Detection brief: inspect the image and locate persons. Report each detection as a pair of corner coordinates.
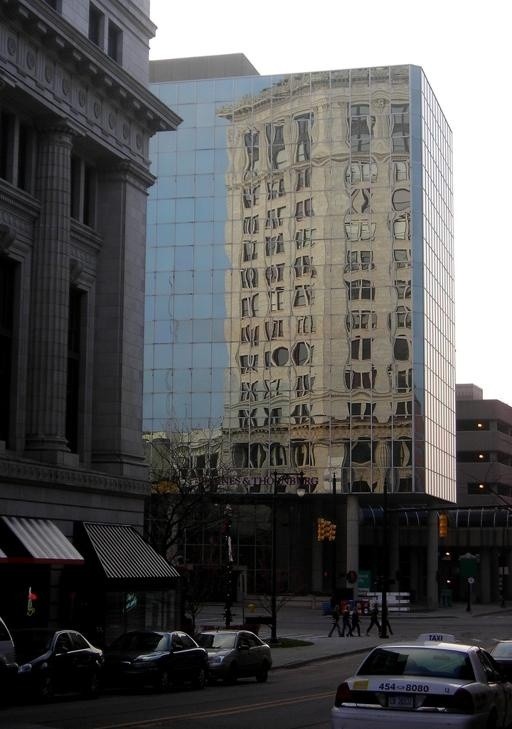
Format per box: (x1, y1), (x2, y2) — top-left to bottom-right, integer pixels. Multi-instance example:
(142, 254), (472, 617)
(366, 602), (382, 636)
(377, 605), (394, 635)
(346, 607), (361, 637)
(341, 604), (353, 637)
(325, 604), (343, 637)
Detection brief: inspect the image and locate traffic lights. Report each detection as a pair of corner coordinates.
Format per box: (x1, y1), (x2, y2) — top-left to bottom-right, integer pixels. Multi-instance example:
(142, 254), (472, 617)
(438, 514), (447, 538)
(325, 520), (332, 538)
(318, 518), (325, 541)
(329, 524), (336, 542)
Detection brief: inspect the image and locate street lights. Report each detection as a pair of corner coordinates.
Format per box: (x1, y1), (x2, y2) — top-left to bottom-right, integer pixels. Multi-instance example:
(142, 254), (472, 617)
(222, 503), (235, 627)
(270, 468), (309, 641)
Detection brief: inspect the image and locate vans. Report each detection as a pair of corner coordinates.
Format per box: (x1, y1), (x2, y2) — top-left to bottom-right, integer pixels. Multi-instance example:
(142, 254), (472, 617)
(0, 616), (16, 662)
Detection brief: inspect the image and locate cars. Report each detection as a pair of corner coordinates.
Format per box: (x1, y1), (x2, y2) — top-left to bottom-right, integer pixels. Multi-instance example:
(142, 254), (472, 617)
(0, 630), (104, 700)
(331, 633), (512, 729)
(193, 630), (272, 685)
(490, 640), (512, 678)
(104, 630), (208, 693)
